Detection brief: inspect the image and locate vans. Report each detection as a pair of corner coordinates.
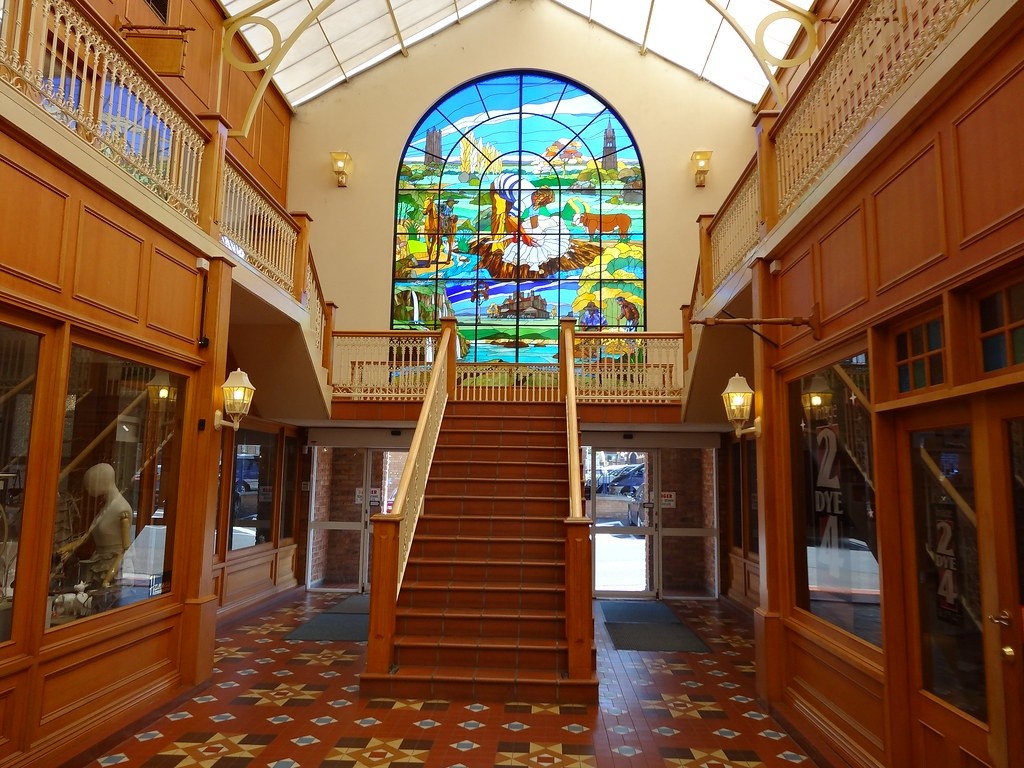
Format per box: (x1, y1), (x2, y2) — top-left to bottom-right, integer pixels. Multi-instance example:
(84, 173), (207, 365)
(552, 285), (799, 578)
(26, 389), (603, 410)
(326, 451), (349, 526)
(608, 462), (645, 497)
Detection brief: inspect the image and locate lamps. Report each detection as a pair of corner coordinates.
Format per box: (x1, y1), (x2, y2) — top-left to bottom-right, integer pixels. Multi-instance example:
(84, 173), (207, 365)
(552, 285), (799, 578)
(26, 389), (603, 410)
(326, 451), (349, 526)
(214, 366), (256, 431)
(721, 372), (761, 440)
(146, 371), (178, 426)
(691, 150), (712, 186)
(800, 374), (834, 429)
(329, 145), (353, 187)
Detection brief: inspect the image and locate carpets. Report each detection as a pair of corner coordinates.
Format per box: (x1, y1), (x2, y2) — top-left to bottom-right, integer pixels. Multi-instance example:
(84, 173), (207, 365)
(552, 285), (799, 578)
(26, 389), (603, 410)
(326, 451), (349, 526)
(281, 614), (370, 641)
(323, 593), (370, 613)
(600, 600), (681, 622)
(604, 622), (713, 652)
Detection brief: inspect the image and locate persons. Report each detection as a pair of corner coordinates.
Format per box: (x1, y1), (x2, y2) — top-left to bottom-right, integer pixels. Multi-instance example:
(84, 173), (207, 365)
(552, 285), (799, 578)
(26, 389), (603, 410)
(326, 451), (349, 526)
(55, 464), (134, 616)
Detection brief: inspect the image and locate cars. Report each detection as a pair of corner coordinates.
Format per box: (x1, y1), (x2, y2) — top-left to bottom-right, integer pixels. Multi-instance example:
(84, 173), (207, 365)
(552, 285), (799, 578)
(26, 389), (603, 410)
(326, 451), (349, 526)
(626, 483), (647, 536)
(217, 454), (259, 521)
